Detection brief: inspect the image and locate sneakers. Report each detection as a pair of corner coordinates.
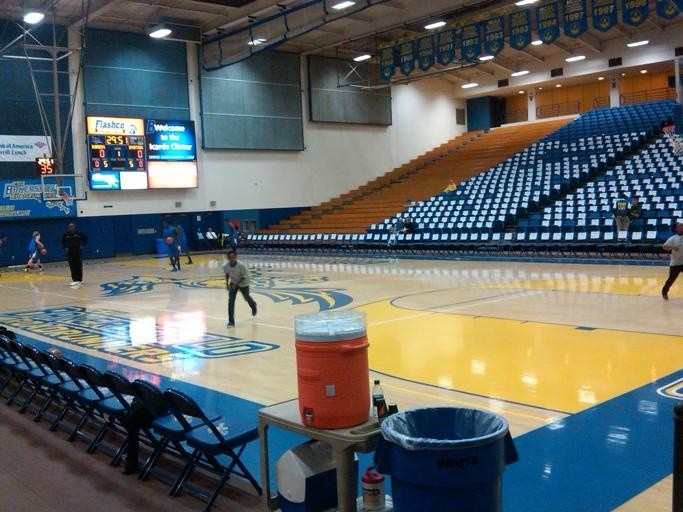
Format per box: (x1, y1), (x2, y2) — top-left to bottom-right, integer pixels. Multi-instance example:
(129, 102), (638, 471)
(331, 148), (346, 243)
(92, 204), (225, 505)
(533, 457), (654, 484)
(227, 321), (235, 328)
(69, 281), (79, 286)
(251, 301), (257, 317)
(661, 290), (669, 300)
(171, 267), (177, 272)
(188, 260), (192, 264)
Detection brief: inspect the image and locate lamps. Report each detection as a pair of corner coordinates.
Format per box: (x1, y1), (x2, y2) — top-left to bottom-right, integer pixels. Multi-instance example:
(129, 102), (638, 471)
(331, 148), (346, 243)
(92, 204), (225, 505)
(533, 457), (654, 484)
(515, 0), (539, 6)
(424, 20), (446, 32)
(145, 4), (173, 40)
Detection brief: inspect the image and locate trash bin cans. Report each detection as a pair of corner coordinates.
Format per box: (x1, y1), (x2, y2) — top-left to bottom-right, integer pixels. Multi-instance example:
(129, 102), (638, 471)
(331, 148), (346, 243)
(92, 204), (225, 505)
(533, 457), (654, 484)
(376, 403), (519, 512)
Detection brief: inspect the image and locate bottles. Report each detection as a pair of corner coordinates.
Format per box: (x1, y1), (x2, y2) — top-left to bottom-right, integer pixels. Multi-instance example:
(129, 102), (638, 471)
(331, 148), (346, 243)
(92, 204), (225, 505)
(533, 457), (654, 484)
(372, 379), (384, 418)
(361, 471), (385, 511)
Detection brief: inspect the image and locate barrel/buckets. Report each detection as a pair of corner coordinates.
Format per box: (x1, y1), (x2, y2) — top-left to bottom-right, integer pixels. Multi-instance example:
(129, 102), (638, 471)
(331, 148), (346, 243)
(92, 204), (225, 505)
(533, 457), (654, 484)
(294, 311), (369, 429)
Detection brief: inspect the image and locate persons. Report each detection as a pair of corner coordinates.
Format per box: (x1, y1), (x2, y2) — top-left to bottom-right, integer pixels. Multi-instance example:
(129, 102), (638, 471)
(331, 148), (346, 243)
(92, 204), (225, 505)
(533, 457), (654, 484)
(196, 218), (241, 251)
(222, 251), (256, 328)
(395, 218), (405, 232)
(443, 180), (457, 193)
(661, 222), (683, 300)
(612, 194), (631, 243)
(22, 231), (44, 272)
(404, 218), (416, 232)
(223, 219), (241, 251)
(161, 220), (193, 272)
(629, 196), (642, 219)
(62, 221), (88, 287)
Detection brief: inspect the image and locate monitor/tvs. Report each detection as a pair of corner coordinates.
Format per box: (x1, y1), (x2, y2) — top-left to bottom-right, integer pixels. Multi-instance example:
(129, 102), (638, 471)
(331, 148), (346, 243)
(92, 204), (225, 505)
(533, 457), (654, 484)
(143, 118), (197, 161)
(148, 161), (200, 189)
(119, 171), (148, 190)
(88, 172), (120, 190)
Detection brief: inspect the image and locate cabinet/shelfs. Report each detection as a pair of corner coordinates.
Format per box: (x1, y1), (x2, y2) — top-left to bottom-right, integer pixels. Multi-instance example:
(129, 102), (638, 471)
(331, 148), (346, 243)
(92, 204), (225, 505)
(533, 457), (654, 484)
(257, 398), (388, 512)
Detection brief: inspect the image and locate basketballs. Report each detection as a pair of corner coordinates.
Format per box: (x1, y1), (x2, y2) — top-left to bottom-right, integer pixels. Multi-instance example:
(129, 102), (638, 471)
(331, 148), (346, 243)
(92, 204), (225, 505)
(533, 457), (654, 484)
(48, 348), (62, 359)
(167, 237), (173, 244)
(41, 249), (46, 255)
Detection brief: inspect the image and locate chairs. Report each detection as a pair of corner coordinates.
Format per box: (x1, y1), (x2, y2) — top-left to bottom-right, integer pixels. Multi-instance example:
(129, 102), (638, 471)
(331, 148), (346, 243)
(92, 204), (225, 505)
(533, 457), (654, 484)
(263, 235), (268, 250)
(345, 234), (351, 252)
(389, 234), (396, 255)
(69, 363), (112, 453)
(100, 369), (162, 487)
(16, 345), (54, 413)
(589, 231), (601, 258)
(616, 230), (629, 259)
(291, 235), (297, 249)
(413, 234), (422, 255)
(257, 234), (262, 249)
(35, 351), (71, 433)
(431, 233), (441, 256)
(127, 378), (220, 502)
(644, 231), (658, 260)
(513, 233), (526, 257)
(337, 234), (344, 251)
(323, 235), (330, 251)
(539, 233), (551, 259)
(6, 339), (45, 403)
(366, 233), (374, 254)
(564, 232), (576, 257)
(280, 235), (285, 249)
(374, 234), (381, 253)
(397, 234), (405, 255)
(441, 234), (449, 255)
(252, 234), (257, 250)
(310, 235), (316, 250)
(316, 234), (323, 251)
(450, 234), (458, 255)
(489, 233), (501, 257)
(359, 233), (366, 254)
(526, 233), (539, 257)
(469, 233), (478, 257)
(162, 389), (264, 509)
(576, 232), (588, 259)
(423, 232), (432, 256)
(459, 233), (469, 256)
(479, 233), (490, 256)
(244, 235), (252, 249)
(0, 335), (31, 398)
(274, 235), (279, 249)
(406, 233), (413, 255)
(269, 235), (274, 250)
(501, 233), (513, 257)
(601, 232), (615, 258)
(352, 234), (359, 253)
(285, 234), (291, 249)
(303, 235), (309, 250)
(297, 235), (303, 250)
(552, 232), (562, 257)
(381, 234), (389, 254)
(331, 234), (337, 251)
(52, 358), (91, 445)
(629, 232), (644, 259)
